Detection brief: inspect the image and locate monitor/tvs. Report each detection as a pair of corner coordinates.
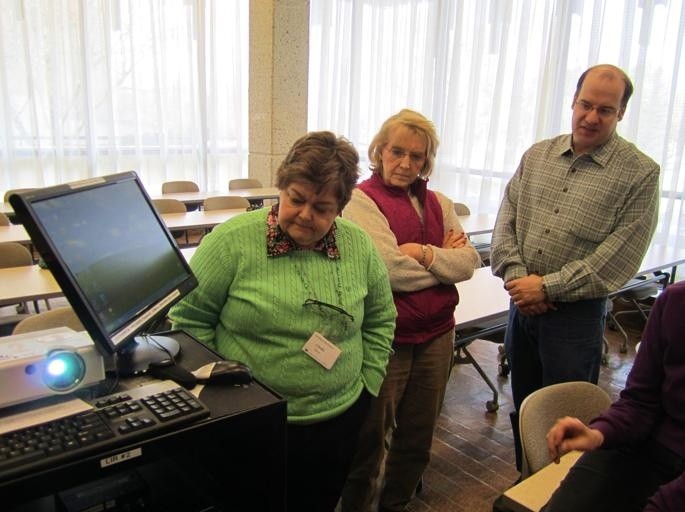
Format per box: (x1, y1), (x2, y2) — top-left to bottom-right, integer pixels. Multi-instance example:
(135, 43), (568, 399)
(0, 325), (108, 407)
(8, 170), (200, 377)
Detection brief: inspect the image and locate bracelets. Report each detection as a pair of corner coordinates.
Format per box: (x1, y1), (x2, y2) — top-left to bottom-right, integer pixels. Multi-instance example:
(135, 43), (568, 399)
(542, 278), (549, 307)
(420, 242), (427, 267)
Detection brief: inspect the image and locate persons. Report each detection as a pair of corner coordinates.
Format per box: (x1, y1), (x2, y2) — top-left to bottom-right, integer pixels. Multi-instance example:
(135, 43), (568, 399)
(490, 64), (660, 472)
(339, 111), (481, 511)
(165, 130), (397, 512)
(538, 280), (684, 511)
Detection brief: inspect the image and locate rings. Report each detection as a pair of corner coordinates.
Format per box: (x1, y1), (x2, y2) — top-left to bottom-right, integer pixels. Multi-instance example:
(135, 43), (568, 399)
(545, 433), (550, 440)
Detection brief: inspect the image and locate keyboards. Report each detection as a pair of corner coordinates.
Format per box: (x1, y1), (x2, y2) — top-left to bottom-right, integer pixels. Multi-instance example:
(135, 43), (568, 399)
(1, 379), (211, 479)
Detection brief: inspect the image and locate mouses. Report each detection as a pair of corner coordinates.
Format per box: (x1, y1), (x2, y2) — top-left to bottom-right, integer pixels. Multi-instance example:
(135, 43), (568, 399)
(192, 358), (254, 386)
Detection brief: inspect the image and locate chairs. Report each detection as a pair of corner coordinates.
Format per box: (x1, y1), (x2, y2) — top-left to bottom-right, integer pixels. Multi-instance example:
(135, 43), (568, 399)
(2, 179), (469, 336)
(519, 382), (631, 480)
(447, 214), (685, 411)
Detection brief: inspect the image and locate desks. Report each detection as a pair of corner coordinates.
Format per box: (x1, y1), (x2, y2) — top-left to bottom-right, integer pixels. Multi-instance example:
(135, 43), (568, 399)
(504, 449), (585, 512)
